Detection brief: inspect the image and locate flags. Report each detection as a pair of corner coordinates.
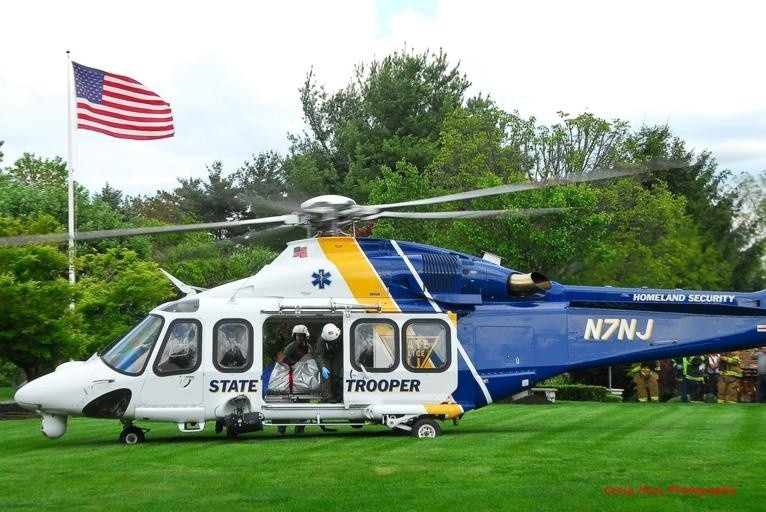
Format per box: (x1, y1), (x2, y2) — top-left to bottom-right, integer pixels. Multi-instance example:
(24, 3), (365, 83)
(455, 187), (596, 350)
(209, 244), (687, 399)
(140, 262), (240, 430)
(72, 61), (176, 141)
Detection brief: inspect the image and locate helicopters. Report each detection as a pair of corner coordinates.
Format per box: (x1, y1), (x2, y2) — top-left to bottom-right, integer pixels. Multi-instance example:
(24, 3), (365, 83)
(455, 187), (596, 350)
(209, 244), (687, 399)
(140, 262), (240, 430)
(0, 151), (766, 444)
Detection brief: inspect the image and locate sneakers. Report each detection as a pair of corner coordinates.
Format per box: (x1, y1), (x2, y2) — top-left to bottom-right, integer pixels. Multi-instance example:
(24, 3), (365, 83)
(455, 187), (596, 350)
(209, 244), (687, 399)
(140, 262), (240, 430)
(278, 429), (303, 434)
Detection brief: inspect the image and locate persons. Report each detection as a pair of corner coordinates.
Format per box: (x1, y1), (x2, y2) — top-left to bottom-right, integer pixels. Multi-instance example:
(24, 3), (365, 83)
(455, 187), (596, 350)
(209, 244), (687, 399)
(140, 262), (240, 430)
(632, 345), (766, 403)
(274, 321), (342, 439)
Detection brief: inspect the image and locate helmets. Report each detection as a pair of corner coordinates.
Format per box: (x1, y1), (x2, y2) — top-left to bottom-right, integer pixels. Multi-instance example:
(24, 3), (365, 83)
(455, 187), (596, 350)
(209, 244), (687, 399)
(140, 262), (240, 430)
(320, 323), (341, 342)
(290, 325), (310, 340)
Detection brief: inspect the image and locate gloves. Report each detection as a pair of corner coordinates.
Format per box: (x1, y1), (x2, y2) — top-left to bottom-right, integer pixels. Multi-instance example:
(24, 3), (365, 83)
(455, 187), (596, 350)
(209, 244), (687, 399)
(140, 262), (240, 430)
(322, 367), (332, 380)
